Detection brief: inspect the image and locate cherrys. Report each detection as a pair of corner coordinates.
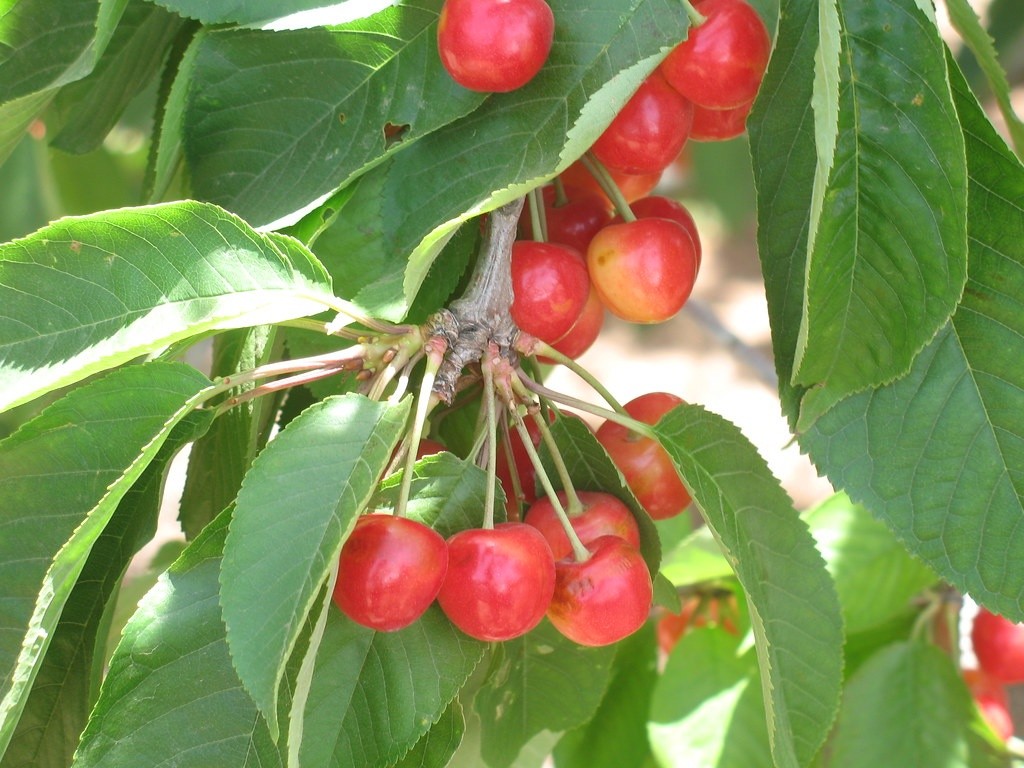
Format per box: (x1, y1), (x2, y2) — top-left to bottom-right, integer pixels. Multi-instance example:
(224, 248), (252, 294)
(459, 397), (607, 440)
(970, 608), (1024, 682)
(962, 670), (1014, 741)
(326, 1), (769, 649)
